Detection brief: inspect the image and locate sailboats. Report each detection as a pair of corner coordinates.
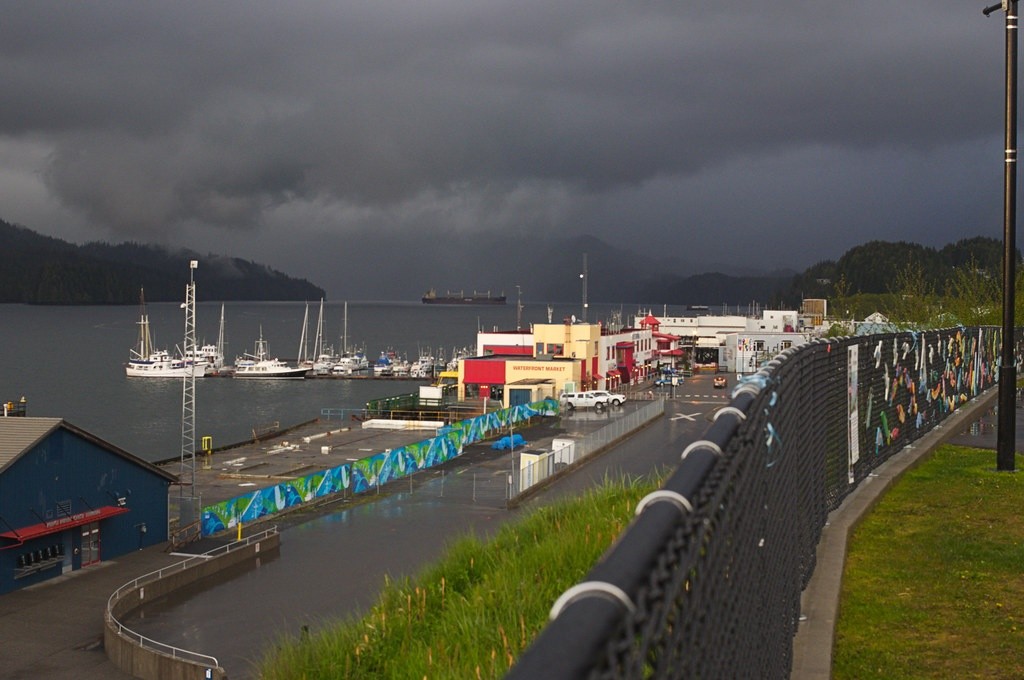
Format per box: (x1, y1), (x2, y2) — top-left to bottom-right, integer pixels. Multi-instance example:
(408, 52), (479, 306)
(125, 288), (229, 378)
(297, 297), (369, 376)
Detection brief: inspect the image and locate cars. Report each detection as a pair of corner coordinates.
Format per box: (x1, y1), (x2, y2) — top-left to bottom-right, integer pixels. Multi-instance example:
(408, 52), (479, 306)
(585, 390), (626, 406)
(713, 376), (727, 387)
(653, 374), (685, 387)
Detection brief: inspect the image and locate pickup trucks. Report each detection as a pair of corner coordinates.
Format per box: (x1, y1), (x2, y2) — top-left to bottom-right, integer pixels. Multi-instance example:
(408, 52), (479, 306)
(559, 393), (609, 409)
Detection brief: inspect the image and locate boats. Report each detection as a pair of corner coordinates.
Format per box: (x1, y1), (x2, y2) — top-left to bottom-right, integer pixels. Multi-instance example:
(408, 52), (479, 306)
(373, 344), (475, 377)
(233, 324), (312, 379)
(422, 289), (508, 304)
